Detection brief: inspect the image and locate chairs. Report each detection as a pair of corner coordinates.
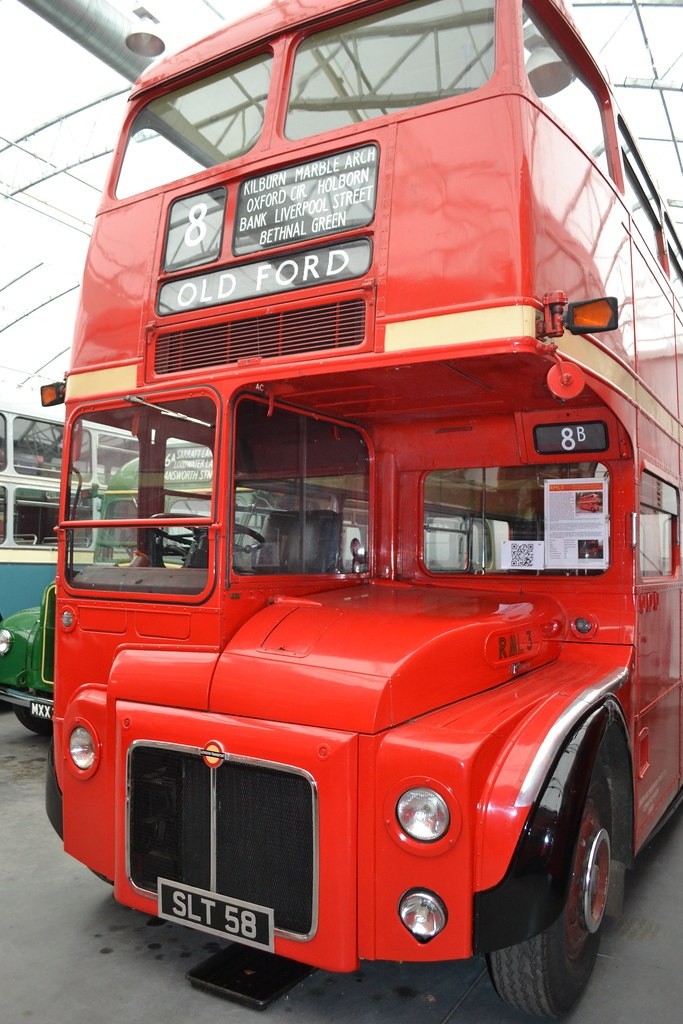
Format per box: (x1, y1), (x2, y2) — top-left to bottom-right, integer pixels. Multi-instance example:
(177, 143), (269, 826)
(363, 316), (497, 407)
(254, 510), (341, 573)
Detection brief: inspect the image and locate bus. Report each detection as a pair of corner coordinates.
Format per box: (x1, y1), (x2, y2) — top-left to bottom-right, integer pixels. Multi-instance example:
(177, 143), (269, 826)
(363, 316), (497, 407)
(42, 1), (683, 1019)
(0, 438), (496, 739)
(0, 409), (137, 621)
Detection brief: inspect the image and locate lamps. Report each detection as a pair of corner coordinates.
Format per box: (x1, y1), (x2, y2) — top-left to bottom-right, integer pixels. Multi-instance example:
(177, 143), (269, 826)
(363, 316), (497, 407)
(125, 14), (166, 58)
